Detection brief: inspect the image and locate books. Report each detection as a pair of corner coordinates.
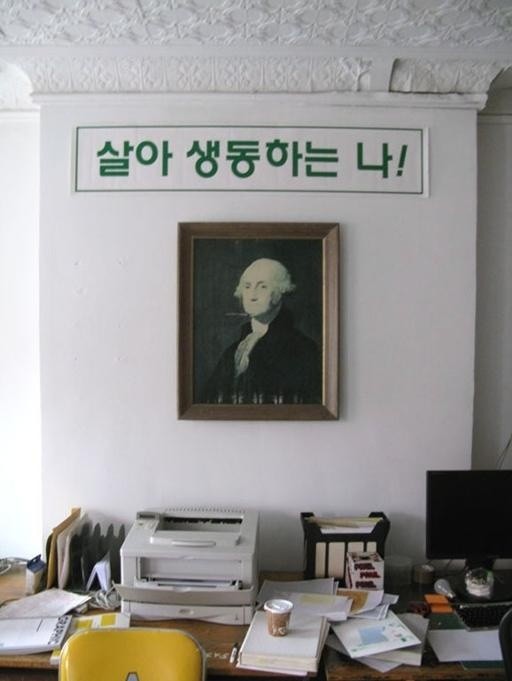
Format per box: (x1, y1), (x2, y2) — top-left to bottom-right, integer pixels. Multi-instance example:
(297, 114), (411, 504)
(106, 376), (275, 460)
(0, 586), (132, 665)
(234, 578), (430, 678)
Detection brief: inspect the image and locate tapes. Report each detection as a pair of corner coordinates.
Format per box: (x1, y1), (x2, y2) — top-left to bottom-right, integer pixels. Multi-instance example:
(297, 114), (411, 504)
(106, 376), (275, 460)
(413, 564), (435, 584)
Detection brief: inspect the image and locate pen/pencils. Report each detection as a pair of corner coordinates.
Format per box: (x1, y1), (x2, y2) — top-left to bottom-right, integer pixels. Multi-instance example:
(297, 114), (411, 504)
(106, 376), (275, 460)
(229, 642), (239, 664)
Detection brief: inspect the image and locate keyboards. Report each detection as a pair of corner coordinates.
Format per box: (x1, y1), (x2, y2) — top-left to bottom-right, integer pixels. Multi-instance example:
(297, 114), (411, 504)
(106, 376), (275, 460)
(456, 604), (512, 631)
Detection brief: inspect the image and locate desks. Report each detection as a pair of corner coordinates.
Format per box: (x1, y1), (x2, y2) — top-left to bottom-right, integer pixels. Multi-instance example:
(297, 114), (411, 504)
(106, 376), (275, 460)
(0, 570), (512, 681)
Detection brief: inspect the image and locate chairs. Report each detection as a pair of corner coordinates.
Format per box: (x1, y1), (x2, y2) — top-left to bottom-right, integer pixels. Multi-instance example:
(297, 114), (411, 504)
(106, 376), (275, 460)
(498, 608), (512, 680)
(57, 626), (206, 681)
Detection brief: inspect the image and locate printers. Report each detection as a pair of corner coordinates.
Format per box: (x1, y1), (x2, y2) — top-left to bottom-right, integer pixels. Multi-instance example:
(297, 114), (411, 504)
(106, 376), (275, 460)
(111, 505), (261, 625)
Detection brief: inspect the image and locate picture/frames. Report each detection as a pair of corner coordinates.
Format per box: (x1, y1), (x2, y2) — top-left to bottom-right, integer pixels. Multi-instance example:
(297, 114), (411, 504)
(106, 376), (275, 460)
(176, 221), (340, 421)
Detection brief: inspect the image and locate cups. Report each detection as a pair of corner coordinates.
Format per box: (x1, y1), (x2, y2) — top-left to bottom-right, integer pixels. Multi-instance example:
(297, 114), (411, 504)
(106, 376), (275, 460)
(264, 600), (293, 636)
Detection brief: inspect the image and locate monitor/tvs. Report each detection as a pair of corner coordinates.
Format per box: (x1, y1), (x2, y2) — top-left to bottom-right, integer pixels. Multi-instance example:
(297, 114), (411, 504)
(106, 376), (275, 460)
(425, 470), (512, 603)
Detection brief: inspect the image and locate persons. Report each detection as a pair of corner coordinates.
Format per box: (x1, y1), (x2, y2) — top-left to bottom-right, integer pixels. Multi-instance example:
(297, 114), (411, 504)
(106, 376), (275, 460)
(201, 257), (322, 405)
